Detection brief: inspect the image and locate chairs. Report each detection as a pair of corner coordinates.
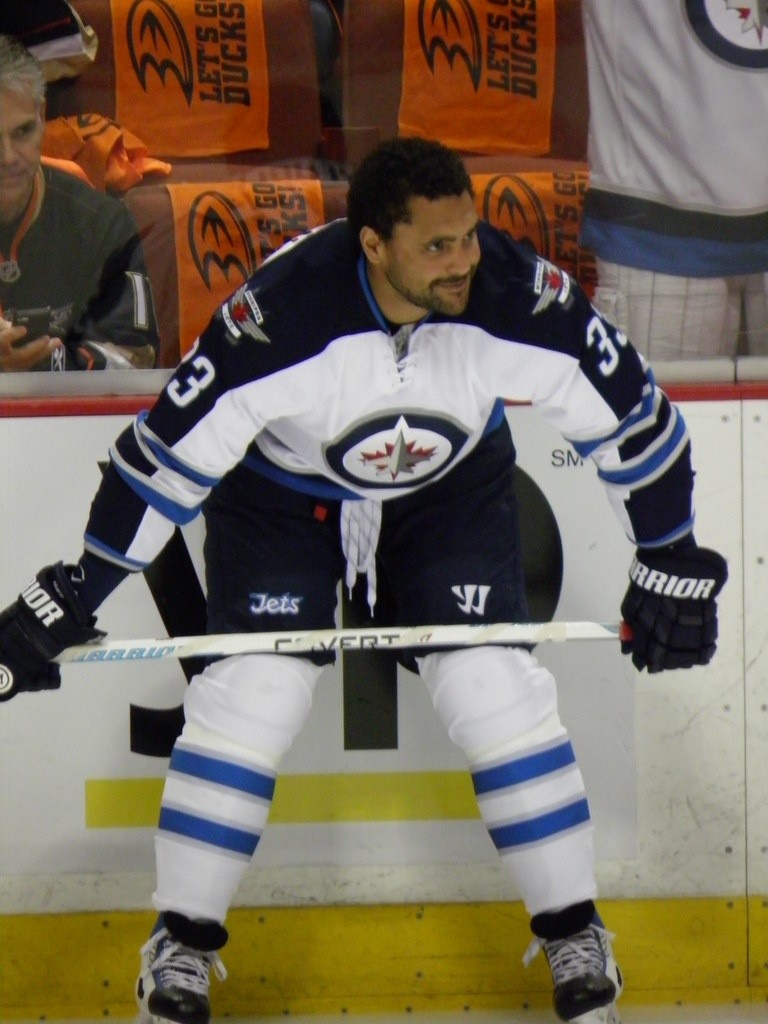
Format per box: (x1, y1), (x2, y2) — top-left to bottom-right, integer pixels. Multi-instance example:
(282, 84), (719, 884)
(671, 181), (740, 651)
(53, 1), (600, 372)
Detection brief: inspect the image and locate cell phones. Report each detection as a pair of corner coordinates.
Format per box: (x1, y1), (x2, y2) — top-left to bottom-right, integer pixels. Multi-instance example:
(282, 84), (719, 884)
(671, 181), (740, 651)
(10, 307), (51, 348)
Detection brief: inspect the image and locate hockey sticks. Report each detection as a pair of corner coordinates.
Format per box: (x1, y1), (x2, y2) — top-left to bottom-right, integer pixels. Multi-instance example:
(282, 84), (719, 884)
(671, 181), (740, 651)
(50, 620), (631, 664)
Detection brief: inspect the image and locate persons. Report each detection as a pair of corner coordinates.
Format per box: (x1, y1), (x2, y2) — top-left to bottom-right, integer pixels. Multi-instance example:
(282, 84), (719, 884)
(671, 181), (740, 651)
(576, 0), (768, 358)
(0, 35), (162, 374)
(0, 131), (730, 1024)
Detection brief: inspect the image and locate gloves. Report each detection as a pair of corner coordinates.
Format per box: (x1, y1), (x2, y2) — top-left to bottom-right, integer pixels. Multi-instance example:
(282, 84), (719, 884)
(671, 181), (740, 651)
(0, 559), (77, 703)
(620, 533), (729, 674)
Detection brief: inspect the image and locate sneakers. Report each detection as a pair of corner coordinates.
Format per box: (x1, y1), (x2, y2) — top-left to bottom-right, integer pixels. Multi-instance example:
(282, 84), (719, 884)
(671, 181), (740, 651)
(134, 910), (228, 1024)
(520, 898), (623, 1024)
